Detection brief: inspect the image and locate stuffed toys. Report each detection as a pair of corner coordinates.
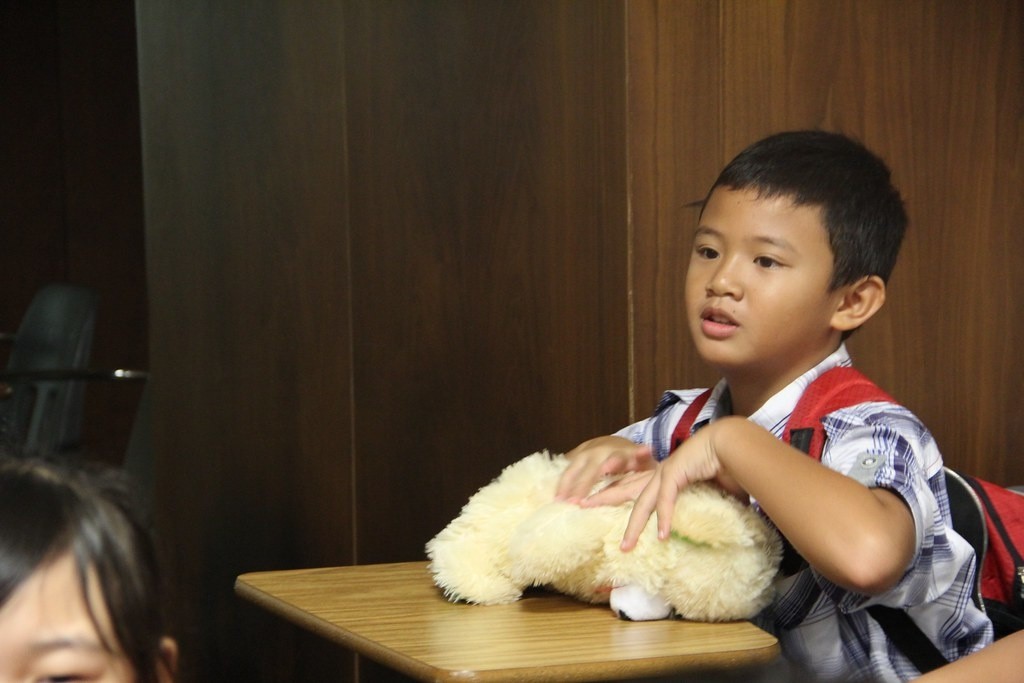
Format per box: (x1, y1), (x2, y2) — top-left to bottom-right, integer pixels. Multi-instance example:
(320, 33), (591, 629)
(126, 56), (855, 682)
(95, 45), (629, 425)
(426, 447), (784, 623)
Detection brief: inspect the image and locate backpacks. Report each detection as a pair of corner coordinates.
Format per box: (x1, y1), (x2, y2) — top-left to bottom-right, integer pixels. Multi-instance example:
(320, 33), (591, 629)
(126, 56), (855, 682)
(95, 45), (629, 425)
(667, 366), (1024, 641)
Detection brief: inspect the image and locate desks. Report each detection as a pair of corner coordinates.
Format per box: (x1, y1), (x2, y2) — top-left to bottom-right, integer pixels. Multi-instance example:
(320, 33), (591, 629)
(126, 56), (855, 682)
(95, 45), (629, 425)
(234, 561), (781, 683)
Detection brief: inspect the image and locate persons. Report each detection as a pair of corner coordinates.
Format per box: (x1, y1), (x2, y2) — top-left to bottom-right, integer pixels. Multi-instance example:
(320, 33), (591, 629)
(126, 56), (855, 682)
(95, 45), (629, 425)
(1, 457), (178, 683)
(557, 130), (993, 683)
